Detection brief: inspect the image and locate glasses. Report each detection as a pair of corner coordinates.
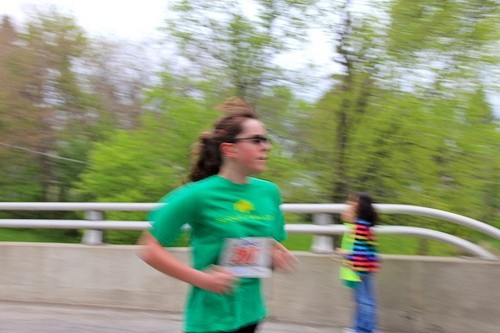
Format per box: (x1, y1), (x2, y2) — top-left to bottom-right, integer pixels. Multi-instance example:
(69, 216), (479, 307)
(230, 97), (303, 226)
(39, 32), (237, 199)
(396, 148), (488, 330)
(231, 134), (269, 144)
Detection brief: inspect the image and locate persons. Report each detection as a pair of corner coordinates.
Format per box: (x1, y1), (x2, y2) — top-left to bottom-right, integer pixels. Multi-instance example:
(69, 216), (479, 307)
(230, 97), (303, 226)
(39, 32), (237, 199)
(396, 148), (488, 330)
(135, 97), (299, 333)
(329, 192), (382, 333)
